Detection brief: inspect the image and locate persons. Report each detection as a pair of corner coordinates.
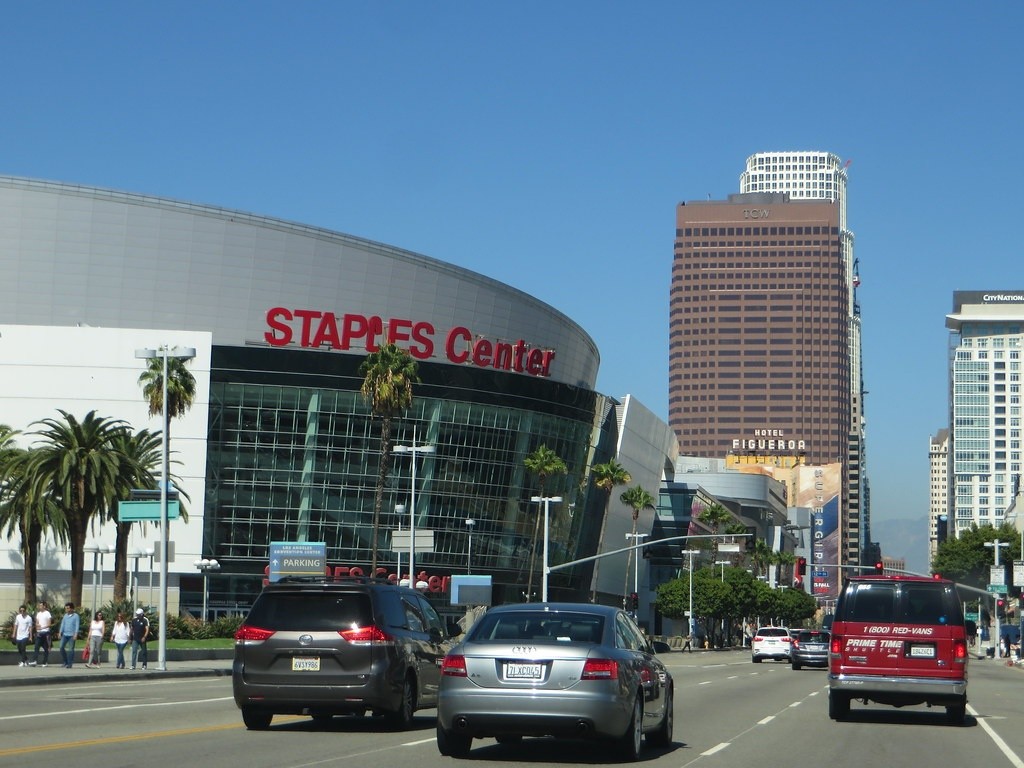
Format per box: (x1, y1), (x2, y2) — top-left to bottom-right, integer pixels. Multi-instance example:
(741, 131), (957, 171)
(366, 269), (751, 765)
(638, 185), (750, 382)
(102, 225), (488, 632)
(29, 602), (51, 666)
(12, 605), (33, 666)
(129, 608), (150, 669)
(1000, 634), (1010, 657)
(86, 611), (105, 668)
(110, 613), (131, 669)
(58, 602), (79, 668)
(682, 633), (692, 653)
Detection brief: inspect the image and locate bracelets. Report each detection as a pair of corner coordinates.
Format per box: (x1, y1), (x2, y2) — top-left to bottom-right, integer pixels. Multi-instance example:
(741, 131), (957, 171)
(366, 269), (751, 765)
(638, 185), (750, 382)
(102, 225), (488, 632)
(144, 636), (146, 638)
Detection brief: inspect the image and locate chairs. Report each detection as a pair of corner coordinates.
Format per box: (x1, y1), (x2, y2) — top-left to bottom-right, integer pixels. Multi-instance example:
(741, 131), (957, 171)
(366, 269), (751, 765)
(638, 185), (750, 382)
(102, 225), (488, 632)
(570, 624), (598, 642)
(498, 623), (520, 640)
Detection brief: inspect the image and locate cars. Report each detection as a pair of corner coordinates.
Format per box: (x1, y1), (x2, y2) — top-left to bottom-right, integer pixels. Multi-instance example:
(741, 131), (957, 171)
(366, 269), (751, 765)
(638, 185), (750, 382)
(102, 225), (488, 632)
(436, 603), (674, 763)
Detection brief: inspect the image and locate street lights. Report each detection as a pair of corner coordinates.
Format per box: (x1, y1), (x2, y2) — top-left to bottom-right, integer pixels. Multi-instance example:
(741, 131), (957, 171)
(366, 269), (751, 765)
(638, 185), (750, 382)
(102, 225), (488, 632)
(193, 558), (221, 627)
(126, 548), (154, 618)
(134, 347), (197, 669)
(83, 546), (116, 621)
(531, 495), (563, 603)
(681, 548), (701, 648)
(983, 538), (1010, 660)
(755, 573), (789, 632)
(714, 559), (733, 648)
(625, 531), (649, 627)
(392, 424), (436, 590)
(465, 518), (477, 575)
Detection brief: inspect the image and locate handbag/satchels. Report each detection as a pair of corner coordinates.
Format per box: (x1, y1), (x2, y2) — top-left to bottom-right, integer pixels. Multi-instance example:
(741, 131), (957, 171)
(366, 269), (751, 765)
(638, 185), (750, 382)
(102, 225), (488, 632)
(82, 645), (89, 660)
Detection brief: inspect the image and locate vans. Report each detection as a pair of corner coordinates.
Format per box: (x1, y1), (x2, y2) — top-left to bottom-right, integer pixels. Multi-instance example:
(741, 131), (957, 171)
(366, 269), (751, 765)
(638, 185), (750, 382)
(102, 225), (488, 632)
(822, 576), (977, 723)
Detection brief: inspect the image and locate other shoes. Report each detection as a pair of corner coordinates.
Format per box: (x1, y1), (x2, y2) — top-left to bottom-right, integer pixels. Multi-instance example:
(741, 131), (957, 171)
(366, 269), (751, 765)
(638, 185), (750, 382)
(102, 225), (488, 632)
(60, 663), (66, 668)
(20, 660), (27, 666)
(129, 666), (136, 670)
(141, 665), (148, 670)
(28, 661), (37, 667)
(93, 664), (101, 669)
(85, 664), (92, 669)
(65, 665), (72, 669)
(41, 663), (49, 668)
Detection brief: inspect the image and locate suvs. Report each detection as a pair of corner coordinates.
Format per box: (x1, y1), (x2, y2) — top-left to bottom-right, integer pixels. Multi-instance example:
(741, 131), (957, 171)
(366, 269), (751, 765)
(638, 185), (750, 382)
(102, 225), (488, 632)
(751, 626), (831, 670)
(231, 576), (463, 730)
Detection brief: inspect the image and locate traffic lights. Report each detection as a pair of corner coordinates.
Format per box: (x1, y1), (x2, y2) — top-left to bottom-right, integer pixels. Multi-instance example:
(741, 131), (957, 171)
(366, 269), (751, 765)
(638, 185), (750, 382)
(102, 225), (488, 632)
(995, 598), (1005, 618)
(875, 561), (884, 574)
(631, 593), (639, 609)
(798, 557), (807, 576)
(933, 573), (943, 579)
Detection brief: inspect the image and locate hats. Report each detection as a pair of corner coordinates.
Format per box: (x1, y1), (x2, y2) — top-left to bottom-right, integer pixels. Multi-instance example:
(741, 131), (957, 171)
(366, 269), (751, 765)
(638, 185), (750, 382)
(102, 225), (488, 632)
(136, 608), (143, 614)
